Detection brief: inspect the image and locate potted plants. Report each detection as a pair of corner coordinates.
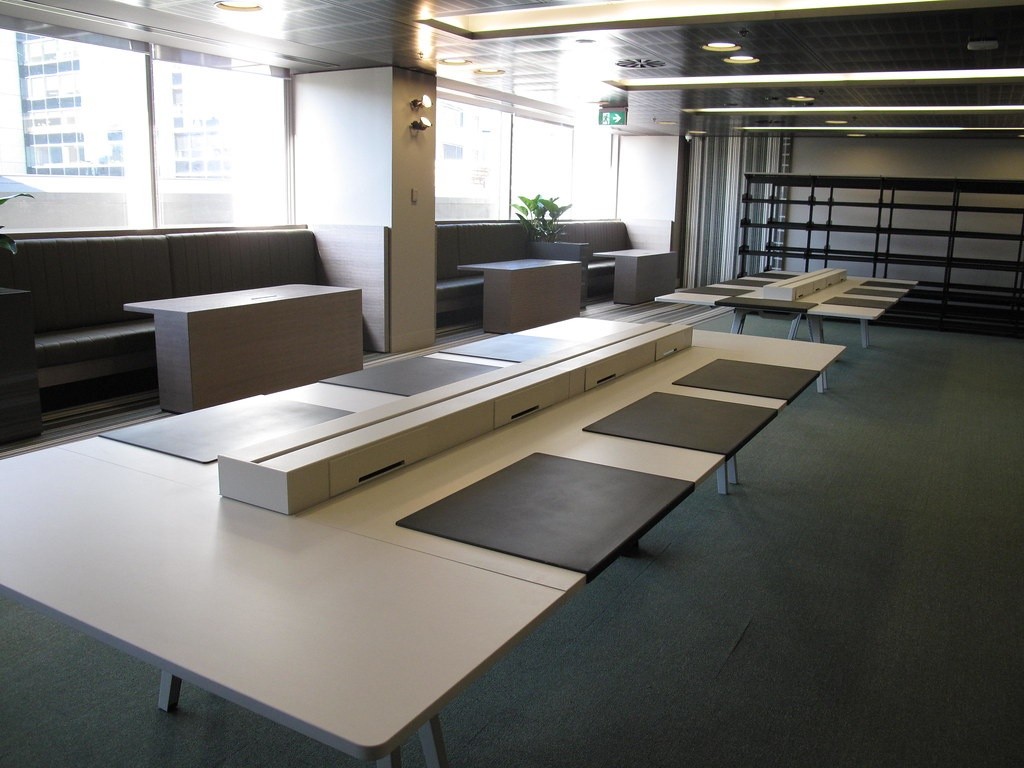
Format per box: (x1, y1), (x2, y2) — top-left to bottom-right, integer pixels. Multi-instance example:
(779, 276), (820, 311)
(517, 195), (569, 242)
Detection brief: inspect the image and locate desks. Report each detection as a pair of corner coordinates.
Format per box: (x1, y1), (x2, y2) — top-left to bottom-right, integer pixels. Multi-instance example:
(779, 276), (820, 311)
(654, 269), (917, 392)
(593, 249), (679, 305)
(456, 259), (583, 334)
(123, 283), (364, 414)
(0, 318), (847, 768)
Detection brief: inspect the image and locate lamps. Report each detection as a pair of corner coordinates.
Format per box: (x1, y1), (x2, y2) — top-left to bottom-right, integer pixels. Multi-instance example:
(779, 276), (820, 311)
(410, 117), (432, 132)
(410, 94), (432, 111)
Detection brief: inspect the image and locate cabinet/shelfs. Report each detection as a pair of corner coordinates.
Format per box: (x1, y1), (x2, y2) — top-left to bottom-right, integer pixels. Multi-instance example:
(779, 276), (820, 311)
(736, 173), (1024, 338)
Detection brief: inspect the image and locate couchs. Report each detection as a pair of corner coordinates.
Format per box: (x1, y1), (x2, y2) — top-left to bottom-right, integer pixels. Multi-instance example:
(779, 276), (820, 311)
(436, 218), (636, 326)
(0, 228), (334, 444)
